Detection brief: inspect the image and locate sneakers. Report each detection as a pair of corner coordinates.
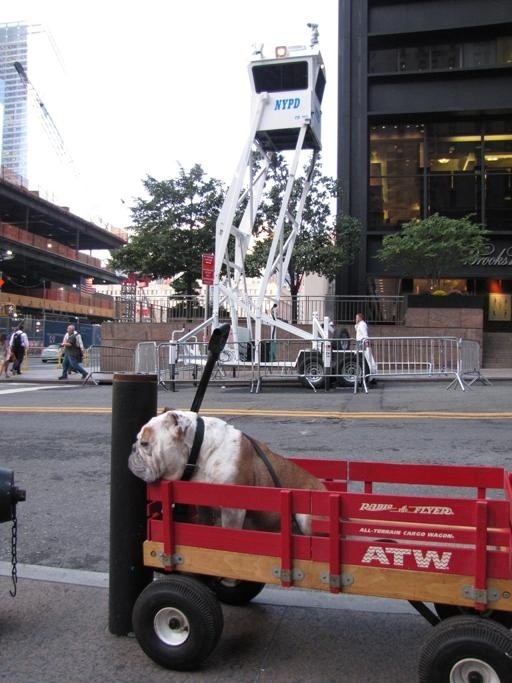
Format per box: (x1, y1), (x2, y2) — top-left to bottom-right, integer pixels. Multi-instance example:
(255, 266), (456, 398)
(58, 376), (68, 380)
(5, 369), (22, 378)
(81, 372), (88, 379)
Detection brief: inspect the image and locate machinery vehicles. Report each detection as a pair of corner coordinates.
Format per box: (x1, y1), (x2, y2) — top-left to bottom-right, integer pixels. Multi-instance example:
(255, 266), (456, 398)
(169, 23), (378, 388)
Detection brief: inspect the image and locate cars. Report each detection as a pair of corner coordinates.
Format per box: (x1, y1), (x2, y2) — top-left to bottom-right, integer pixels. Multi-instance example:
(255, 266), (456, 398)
(41, 343), (64, 362)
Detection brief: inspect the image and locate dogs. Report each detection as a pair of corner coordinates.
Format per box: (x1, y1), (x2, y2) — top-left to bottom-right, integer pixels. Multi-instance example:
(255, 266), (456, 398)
(126, 410), (352, 539)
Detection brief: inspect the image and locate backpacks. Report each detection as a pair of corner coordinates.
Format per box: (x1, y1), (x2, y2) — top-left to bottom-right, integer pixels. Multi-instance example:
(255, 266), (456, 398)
(11, 332), (23, 349)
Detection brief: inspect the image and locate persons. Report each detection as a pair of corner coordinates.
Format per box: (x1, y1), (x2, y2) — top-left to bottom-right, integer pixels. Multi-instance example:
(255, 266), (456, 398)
(0, 333), (11, 378)
(62, 330), (84, 375)
(9, 325), (29, 376)
(354, 313), (378, 385)
(57, 324), (89, 380)
